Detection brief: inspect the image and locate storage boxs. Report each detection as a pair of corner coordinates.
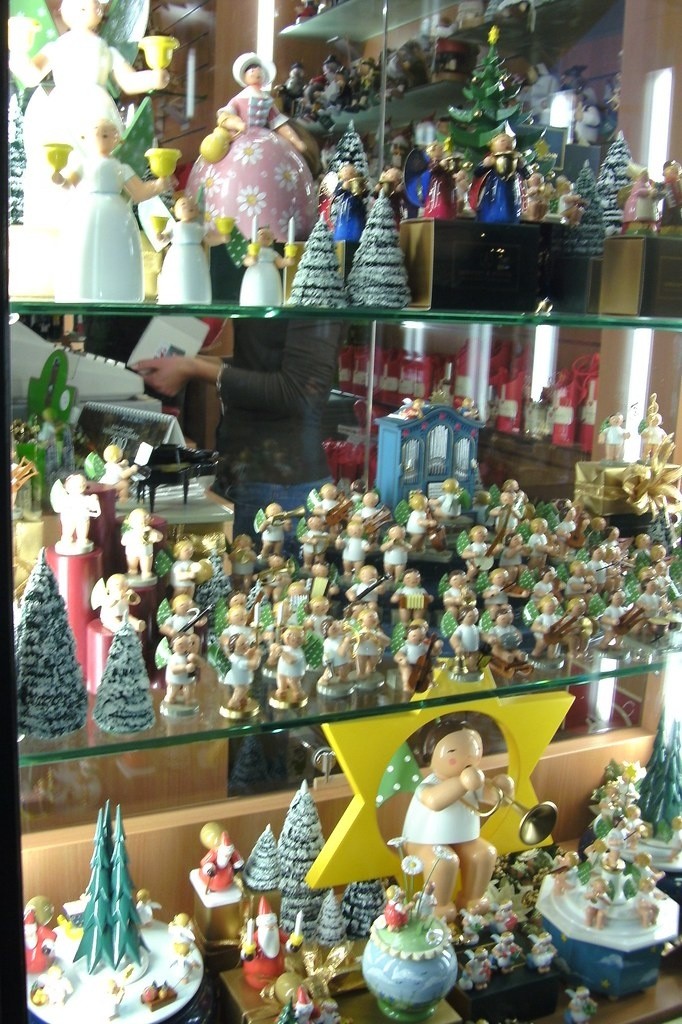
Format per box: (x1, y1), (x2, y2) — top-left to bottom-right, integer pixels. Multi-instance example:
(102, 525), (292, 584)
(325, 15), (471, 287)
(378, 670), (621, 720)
(519, 121), (568, 171)
(393, 215), (540, 311)
(597, 232), (680, 317)
(554, 145), (601, 179)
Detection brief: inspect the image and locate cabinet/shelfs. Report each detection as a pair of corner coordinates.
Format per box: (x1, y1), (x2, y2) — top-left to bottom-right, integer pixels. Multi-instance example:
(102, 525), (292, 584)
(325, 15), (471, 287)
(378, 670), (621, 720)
(277, 1), (560, 131)
(8, 295), (682, 1023)
(480, 428), (588, 507)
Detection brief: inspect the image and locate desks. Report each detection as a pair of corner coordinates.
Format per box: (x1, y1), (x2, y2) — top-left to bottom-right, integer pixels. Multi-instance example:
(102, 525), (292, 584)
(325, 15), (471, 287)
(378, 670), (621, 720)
(115, 489), (236, 533)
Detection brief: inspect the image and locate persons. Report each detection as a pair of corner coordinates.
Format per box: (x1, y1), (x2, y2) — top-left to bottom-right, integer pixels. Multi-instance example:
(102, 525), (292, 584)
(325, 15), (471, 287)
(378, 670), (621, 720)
(598, 410), (630, 460)
(568, 986), (598, 1024)
(197, 828), (244, 892)
(444, 897), (557, 990)
(151, 196), (231, 305)
(324, 130), (682, 244)
(640, 412), (669, 463)
(7, 0), (169, 146)
(130, 316), (343, 793)
(184, 52), (319, 244)
(49, 108), (171, 303)
(274, 0), (604, 180)
(10, 482), (682, 721)
(97, 444), (139, 503)
(400, 721), (516, 942)
(24, 888), (201, 1020)
(547, 763), (682, 931)
(239, 227), (294, 307)
(241, 894), (303, 988)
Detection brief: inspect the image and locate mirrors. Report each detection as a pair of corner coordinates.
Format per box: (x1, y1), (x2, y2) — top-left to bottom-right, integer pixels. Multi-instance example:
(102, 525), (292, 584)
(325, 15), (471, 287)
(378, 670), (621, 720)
(6, 0), (681, 898)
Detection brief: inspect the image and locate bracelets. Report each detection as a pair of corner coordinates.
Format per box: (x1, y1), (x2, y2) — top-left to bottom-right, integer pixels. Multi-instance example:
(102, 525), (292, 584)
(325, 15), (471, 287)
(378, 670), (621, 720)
(216, 364), (231, 415)
